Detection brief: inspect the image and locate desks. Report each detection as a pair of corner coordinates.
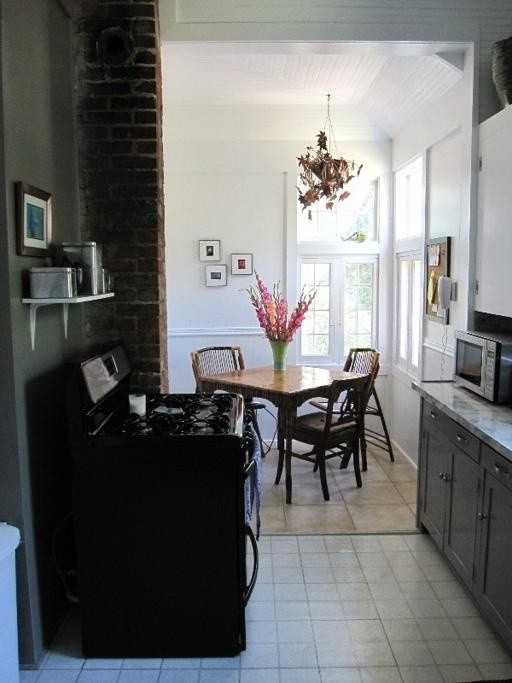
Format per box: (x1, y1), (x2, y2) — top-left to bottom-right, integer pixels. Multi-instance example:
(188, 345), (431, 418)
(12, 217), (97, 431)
(201, 366), (370, 504)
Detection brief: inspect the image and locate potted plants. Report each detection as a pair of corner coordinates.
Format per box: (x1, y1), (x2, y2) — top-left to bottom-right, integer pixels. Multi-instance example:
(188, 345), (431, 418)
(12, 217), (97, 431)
(295, 130), (363, 220)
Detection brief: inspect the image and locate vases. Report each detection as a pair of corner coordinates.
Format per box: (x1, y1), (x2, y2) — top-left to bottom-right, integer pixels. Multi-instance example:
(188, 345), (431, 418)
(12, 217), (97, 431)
(269, 342), (291, 372)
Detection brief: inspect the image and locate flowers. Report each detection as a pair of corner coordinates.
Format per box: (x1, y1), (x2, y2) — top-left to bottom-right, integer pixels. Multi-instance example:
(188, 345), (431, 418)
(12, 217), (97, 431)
(238, 269), (318, 342)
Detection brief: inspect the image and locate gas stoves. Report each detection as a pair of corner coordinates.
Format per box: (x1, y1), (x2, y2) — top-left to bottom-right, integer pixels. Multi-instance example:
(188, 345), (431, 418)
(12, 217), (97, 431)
(98, 392), (243, 439)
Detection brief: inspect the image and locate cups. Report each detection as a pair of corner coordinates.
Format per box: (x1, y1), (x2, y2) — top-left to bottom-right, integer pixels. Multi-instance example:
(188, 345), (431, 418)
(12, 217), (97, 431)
(126, 394), (147, 416)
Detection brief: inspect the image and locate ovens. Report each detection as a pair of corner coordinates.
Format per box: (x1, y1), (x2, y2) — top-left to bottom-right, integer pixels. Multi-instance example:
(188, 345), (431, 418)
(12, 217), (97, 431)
(239, 405), (262, 613)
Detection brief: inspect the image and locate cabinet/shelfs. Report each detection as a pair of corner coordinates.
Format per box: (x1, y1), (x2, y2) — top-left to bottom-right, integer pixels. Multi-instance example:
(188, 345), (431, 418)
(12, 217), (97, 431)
(415, 396), (512, 657)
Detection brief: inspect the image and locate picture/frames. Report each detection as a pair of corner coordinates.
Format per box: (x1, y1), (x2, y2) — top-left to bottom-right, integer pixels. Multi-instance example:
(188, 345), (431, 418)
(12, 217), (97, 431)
(206, 264), (228, 288)
(231, 253), (253, 275)
(15, 181), (53, 258)
(199, 240), (220, 261)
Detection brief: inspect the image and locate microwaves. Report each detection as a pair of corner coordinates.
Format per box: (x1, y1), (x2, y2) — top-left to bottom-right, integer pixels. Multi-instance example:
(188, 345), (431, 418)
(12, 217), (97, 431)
(452, 328), (511, 402)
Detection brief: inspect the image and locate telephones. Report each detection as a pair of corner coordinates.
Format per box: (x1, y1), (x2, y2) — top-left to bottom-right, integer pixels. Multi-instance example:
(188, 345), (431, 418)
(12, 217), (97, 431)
(438, 274), (452, 310)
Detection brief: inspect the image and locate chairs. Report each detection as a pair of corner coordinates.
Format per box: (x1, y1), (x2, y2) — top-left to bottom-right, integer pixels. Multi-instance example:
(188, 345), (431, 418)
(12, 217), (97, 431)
(191, 345), (266, 459)
(309, 348), (395, 472)
(274, 374), (372, 501)
